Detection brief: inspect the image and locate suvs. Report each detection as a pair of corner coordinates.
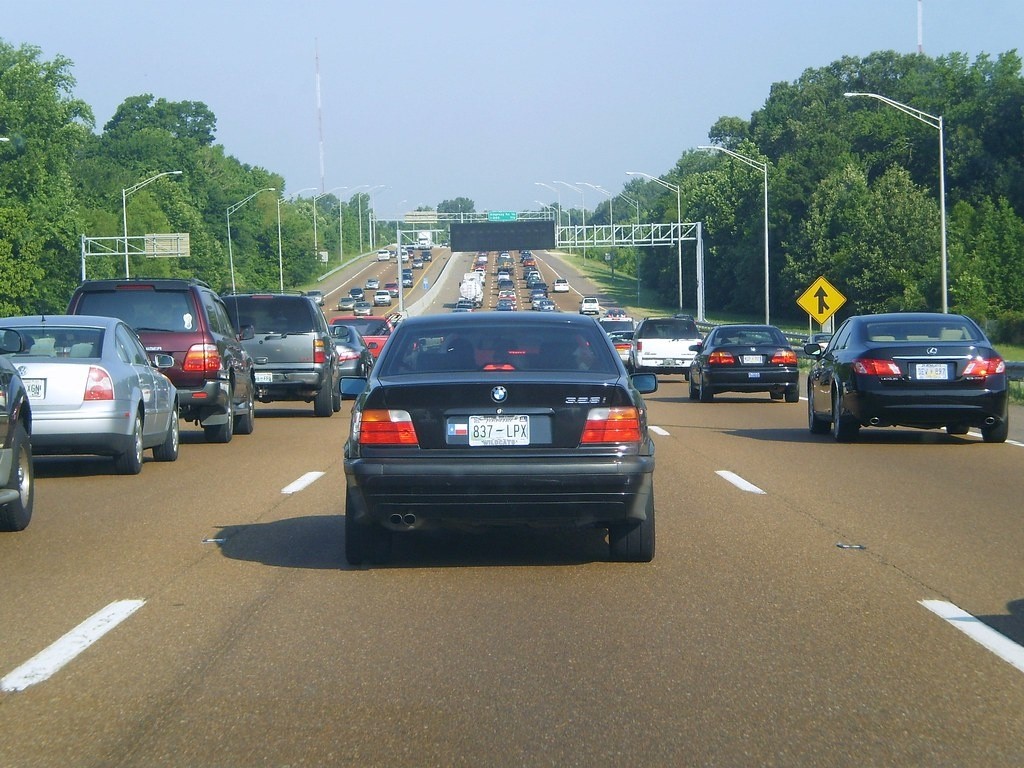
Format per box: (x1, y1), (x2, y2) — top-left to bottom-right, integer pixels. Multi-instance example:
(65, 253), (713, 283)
(65, 277), (258, 444)
(218, 290), (343, 418)
(621, 314), (705, 382)
(384, 282), (399, 298)
(347, 287), (366, 302)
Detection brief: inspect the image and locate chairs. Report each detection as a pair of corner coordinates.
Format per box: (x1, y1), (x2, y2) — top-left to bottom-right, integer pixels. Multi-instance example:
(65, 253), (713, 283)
(720, 339), (728, 344)
(416, 351), (449, 371)
(873, 329), (963, 341)
(446, 338), (476, 370)
(645, 329), (658, 338)
(545, 355), (576, 370)
(30, 344), (57, 358)
(366, 324), (383, 335)
(70, 343), (93, 358)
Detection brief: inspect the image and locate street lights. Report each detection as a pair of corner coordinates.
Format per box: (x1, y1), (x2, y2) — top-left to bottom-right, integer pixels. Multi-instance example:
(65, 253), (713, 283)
(696, 145), (770, 325)
(226, 188), (276, 293)
(535, 181), (640, 226)
(122, 170), (183, 280)
(313, 186), (348, 259)
(340, 184), (392, 260)
(625, 171), (683, 311)
(843, 92), (948, 313)
(277, 187), (318, 293)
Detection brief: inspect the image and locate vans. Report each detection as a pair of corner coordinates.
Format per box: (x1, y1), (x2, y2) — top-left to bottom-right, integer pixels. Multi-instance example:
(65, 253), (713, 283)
(373, 290), (392, 307)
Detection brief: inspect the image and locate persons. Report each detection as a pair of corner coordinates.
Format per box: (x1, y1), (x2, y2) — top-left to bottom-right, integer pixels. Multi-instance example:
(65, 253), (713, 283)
(54, 338), (70, 358)
(448, 340), (476, 368)
(21, 335), (35, 354)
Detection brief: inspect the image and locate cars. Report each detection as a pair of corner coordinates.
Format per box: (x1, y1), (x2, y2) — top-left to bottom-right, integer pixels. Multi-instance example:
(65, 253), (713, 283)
(578, 297), (599, 315)
(596, 308), (636, 368)
(328, 316), (397, 361)
(439, 239), (449, 247)
(0, 315), (181, 475)
(801, 332), (834, 350)
(0, 327), (36, 534)
(804, 312), (1010, 443)
(453, 250), (556, 312)
(352, 302), (373, 316)
(552, 278), (570, 292)
(375, 232), (433, 288)
(687, 324), (801, 405)
(337, 297), (357, 311)
(338, 310), (661, 564)
(286, 290), (325, 306)
(365, 278), (381, 289)
(327, 324), (379, 401)
